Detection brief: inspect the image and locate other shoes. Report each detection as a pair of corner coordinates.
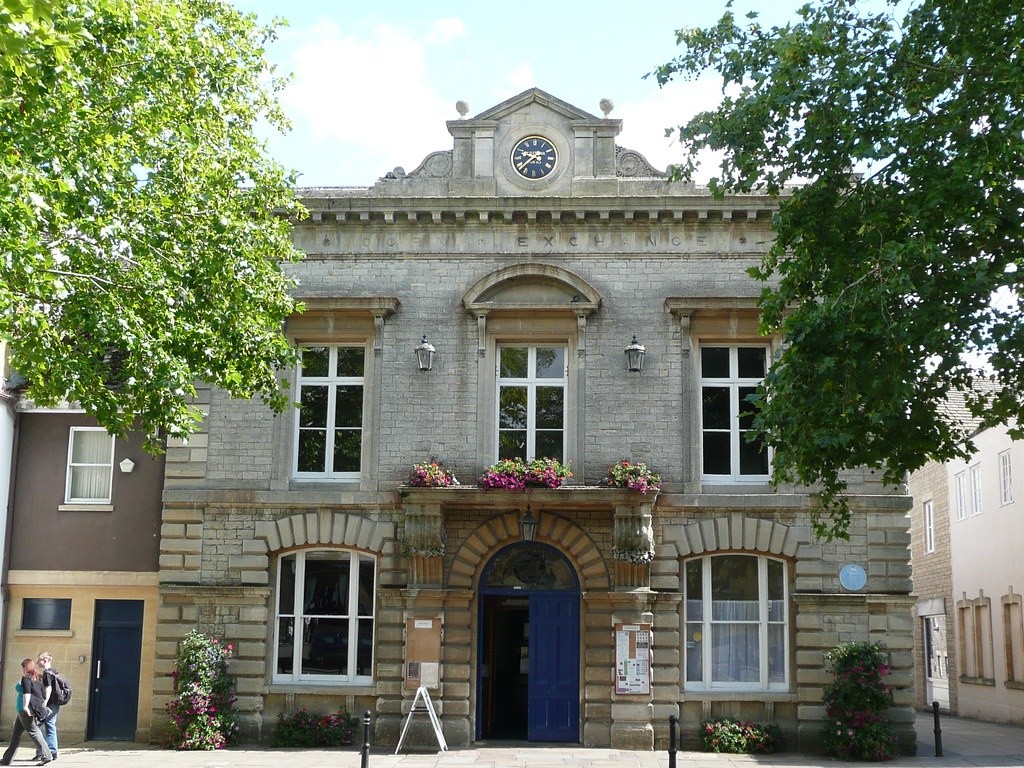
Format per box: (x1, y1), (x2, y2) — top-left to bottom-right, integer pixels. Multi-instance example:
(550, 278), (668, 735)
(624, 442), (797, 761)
(0, 759), (10, 766)
(36, 759), (51, 766)
(32, 753), (58, 760)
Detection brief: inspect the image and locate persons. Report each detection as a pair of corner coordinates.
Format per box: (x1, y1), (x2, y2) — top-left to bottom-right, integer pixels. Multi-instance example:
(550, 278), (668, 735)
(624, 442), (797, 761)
(0, 659), (54, 766)
(31, 651), (61, 762)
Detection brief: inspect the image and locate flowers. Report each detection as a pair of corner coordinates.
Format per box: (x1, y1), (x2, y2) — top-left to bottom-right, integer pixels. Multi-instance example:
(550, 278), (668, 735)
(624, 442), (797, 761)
(473, 453), (576, 493)
(162, 626), (241, 751)
(268, 705), (360, 750)
(701, 716), (786, 756)
(595, 458), (662, 496)
(817, 639), (903, 762)
(401, 455), (461, 488)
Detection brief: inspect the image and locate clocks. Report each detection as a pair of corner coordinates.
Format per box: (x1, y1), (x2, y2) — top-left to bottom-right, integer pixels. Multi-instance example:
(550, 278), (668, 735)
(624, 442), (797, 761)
(498, 126), (572, 192)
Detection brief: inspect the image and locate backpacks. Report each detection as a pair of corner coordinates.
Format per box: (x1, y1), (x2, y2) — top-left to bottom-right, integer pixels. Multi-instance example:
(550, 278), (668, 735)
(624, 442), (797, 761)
(42, 671), (72, 706)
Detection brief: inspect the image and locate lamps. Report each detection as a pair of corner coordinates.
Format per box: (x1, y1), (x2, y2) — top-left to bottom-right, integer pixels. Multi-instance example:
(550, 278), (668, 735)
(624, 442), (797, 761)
(623, 334), (647, 374)
(412, 333), (435, 372)
